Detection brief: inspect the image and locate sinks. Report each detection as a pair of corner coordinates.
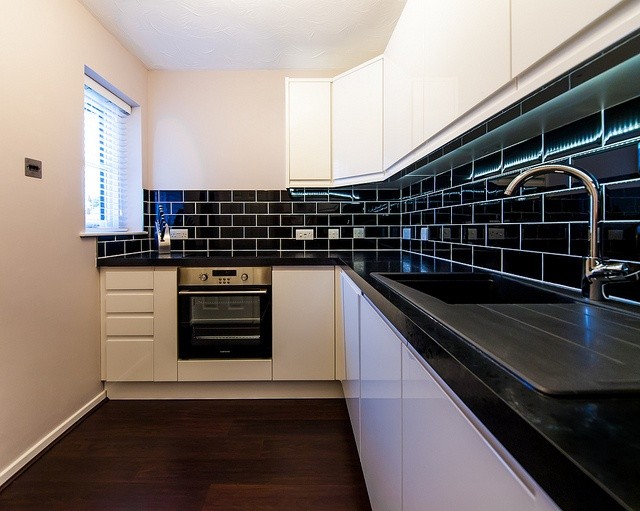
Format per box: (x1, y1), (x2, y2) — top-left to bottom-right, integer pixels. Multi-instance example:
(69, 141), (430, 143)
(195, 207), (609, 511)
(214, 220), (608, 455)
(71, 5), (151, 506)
(380, 267), (576, 308)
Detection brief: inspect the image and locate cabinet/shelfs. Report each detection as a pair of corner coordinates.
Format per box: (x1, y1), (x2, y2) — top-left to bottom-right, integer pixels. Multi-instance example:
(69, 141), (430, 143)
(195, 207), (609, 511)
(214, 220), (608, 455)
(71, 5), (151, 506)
(271, 266), (335, 380)
(105, 271), (178, 382)
(332, 56), (383, 180)
(383, 1), (425, 174)
(285, 75), (333, 187)
(402, 343), (560, 511)
(424, 2), (511, 140)
(511, 0), (640, 77)
(342, 277), (402, 510)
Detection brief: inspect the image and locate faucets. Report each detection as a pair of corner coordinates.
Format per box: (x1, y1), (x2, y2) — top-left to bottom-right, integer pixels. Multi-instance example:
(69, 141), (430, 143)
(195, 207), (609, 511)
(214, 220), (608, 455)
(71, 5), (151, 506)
(503, 160), (601, 254)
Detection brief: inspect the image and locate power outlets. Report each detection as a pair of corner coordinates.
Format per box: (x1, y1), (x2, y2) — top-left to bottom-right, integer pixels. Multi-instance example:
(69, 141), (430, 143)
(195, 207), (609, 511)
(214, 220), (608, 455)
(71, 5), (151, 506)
(295, 229), (314, 241)
(353, 227), (366, 240)
(328, 229), (339, 241)
(402, 227), (411, 241)
(440, 227), (452, 242)
(488, 227), (505, 241)
(467, 227), (478, 240)
(169, 228), (188, 240)
(419, 227), (430, 241)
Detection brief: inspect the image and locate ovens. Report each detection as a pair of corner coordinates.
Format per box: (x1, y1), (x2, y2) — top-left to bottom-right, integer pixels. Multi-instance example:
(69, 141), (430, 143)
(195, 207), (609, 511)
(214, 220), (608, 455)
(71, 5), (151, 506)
(177, 267), (271, 359)
